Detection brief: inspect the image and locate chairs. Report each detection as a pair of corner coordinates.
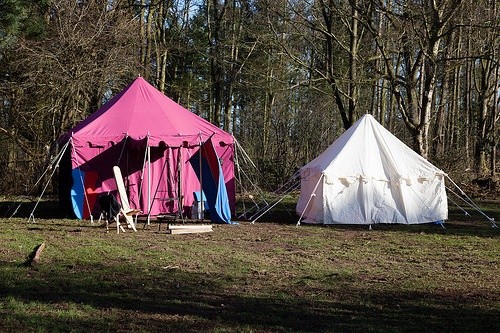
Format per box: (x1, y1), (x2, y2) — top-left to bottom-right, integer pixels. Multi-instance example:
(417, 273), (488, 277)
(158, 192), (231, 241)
(156, 198), (179, 234)
(98, 194), (141, 234)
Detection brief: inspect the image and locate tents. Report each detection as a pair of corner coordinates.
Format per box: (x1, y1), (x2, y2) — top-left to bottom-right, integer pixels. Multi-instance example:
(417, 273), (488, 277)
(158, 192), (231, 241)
(5, 74), (292, 227)
(236, 110), (499, 230)
